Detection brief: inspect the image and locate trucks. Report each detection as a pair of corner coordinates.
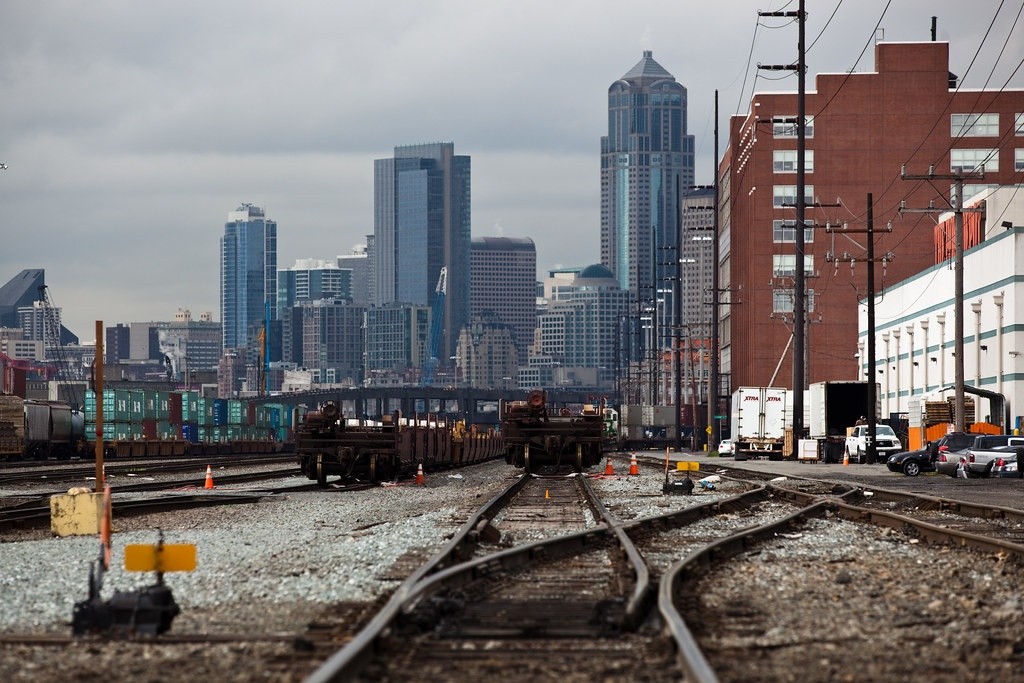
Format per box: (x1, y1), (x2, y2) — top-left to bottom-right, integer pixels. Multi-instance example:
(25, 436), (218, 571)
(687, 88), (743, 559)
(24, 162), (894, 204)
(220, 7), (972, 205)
(729, 386), (786, 462)
(808, 380), (882, 465)
(797, 439), (819, 464)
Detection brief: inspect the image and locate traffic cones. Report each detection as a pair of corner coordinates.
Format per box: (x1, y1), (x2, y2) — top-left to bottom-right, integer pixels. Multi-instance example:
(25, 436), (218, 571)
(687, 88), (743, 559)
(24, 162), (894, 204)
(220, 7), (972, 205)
(413, 464), (425, 485)
(627, 451), (641, 475)
(603, 455), (618, 476)
(842, 452), (849, 466)
(204, 464), (215, 489)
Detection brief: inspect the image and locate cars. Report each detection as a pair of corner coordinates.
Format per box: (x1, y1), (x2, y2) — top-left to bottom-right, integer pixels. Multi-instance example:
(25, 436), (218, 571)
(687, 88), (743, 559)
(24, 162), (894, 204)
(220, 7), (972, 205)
(886, 439), (943, 476)
(718, 439), (735, 457)
(989, 454), (1023, 479)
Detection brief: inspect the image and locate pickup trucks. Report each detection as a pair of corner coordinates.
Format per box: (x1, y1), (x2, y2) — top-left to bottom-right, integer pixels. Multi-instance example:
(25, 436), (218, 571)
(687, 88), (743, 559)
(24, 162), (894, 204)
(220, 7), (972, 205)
(845, 424), (903, 465)
(966, 435), (1024, 479)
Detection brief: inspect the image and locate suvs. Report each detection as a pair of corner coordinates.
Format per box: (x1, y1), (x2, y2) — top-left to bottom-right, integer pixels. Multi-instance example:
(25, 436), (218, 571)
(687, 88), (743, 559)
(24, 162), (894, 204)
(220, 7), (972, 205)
(932, 431), (984, 479)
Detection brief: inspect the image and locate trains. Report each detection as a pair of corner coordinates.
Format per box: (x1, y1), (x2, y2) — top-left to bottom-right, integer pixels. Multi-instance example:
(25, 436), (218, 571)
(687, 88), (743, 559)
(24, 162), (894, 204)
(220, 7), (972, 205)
(21, 401), (294, 461)
(292, 404), (504, 486)
(498, 385), (605, 474)
